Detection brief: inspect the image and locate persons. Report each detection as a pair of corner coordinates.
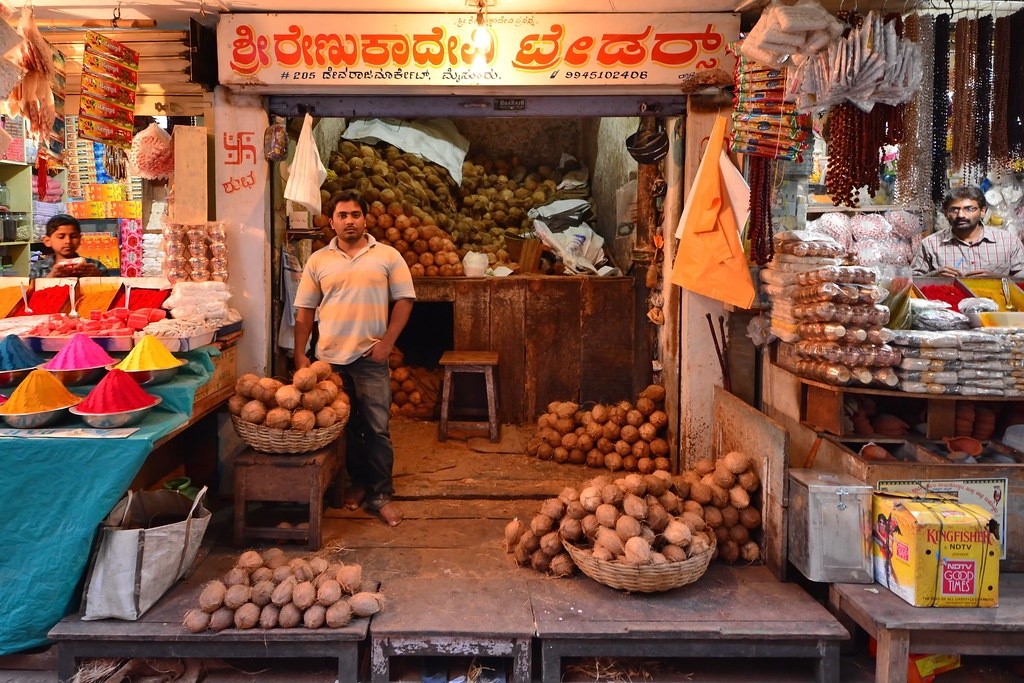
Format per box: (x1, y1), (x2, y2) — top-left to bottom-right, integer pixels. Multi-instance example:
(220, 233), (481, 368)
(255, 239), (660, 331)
(293, 189), (416, 526)
(29, 214), (110, 278)
(907, 182), (1024, 280)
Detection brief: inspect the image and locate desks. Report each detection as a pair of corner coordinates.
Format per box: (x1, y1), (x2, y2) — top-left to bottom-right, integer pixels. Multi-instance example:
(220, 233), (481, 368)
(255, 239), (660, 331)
(0, 330), (243, 612)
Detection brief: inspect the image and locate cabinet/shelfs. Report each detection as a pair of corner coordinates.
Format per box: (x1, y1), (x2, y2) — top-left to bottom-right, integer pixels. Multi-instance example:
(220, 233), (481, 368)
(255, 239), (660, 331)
(0, 160), (68, 277)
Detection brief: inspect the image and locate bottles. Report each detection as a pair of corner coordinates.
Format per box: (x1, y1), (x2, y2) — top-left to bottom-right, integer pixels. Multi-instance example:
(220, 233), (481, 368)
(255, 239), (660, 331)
(0, 183), (30, 244)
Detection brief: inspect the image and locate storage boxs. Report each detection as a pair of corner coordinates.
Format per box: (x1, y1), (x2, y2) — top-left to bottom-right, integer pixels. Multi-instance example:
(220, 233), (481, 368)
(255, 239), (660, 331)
(788, 468), (874, 584)
(0, 278), (244, 350)
(870, 489), (1001, 608)
(911, 275), (1024, 327)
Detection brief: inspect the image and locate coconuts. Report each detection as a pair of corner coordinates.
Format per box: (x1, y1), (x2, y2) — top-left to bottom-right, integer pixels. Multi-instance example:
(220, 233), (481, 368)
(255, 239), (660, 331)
(385, 344), (436, 419)
(185, 521), (385, 629)
(312, 141), (583, 277)
(525, 385), (672, 473)
(226, 359), (352, 430)
(506, 452), (761, 573)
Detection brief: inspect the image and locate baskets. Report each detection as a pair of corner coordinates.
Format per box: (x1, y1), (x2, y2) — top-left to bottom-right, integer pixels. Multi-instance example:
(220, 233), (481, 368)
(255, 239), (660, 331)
(230, 405), (352, 455)
(559, 525), (717, 594)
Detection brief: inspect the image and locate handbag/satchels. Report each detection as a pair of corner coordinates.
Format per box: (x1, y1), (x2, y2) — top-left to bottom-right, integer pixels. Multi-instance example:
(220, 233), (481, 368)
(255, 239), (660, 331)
(78, 485), (212, 621)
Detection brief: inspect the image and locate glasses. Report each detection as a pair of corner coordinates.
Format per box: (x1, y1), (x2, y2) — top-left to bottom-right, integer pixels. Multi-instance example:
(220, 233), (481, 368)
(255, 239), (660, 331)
(947, 206), (980, 214)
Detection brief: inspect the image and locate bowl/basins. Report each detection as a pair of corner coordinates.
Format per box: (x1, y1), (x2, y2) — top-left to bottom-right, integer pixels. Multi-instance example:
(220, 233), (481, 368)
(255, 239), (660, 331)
(104, 359), (188, 387)
(0, 358), (51, 389)
(0, 393), (85, 428)
(68, 394), (163, 428)
(842, 395), (996, 458)
(36, 358), (120, 387)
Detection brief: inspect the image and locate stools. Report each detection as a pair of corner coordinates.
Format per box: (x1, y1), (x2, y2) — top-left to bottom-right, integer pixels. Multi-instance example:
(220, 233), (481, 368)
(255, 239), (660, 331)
(438, 352), (501, 443)
(233, 429), (346, 552)
